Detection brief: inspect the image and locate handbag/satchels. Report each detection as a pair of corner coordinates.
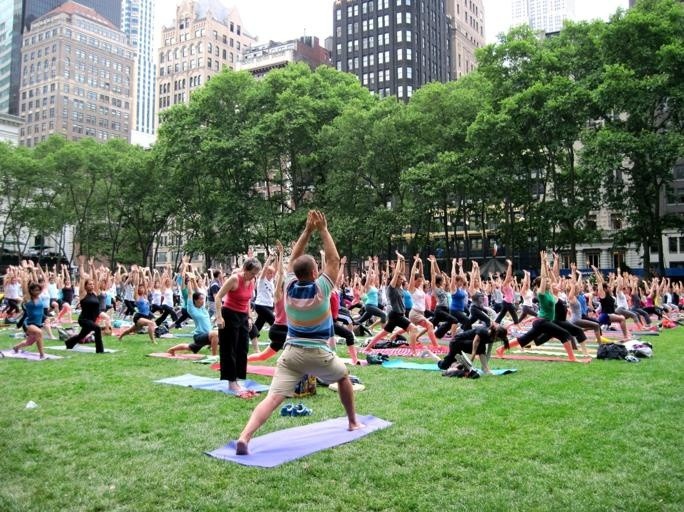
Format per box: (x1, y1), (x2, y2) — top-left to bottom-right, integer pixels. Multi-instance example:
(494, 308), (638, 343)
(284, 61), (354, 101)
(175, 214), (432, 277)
(597, 343), (627, 361)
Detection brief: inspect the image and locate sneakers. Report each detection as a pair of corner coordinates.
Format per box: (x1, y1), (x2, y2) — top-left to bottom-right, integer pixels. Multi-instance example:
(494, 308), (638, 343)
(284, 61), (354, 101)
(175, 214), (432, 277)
(290, 403), (312, 416)
(280, 403), (293, 416)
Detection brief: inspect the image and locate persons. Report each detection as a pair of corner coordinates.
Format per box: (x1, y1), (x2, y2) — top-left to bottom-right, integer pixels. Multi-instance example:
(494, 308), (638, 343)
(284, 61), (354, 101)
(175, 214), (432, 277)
(1, 248), (278, 360)
(353, 250), (684, 361)
(420, 325), (510, 375)
(215, 258), (261, 390)
(236, 210), (366, 456)
